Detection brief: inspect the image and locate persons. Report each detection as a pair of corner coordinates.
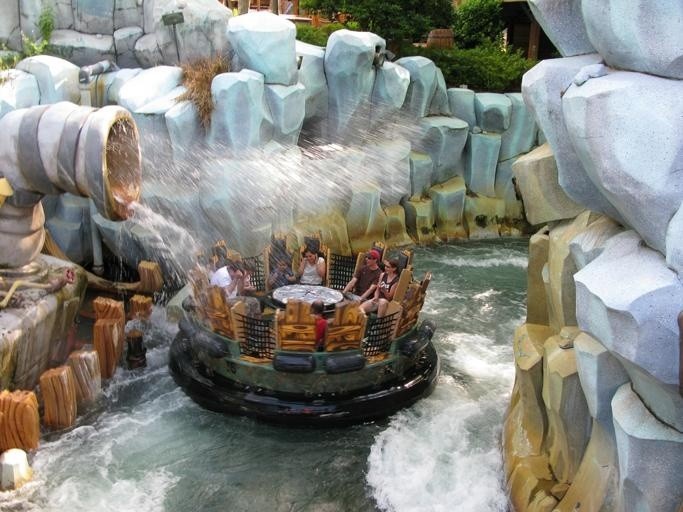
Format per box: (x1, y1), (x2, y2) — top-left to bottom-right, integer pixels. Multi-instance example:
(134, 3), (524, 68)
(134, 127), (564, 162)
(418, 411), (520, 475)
(341, 248), (384, 304)
(268, 256), (296, 289)
(308, 300), (329, 352)
(209, 262), (262, 322)
(239, 262), (258, 297)
(356, 258), (400, 344)
(294, 245), (326, 286)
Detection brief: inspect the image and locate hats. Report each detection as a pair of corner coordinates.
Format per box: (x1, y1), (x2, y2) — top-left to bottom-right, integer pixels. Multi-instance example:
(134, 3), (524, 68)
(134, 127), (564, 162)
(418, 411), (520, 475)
(363, 250), (380, 260)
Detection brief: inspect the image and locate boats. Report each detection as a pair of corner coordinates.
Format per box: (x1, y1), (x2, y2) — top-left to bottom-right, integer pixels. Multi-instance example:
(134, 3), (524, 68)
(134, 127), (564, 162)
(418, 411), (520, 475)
(166, 230), (442, 430)
